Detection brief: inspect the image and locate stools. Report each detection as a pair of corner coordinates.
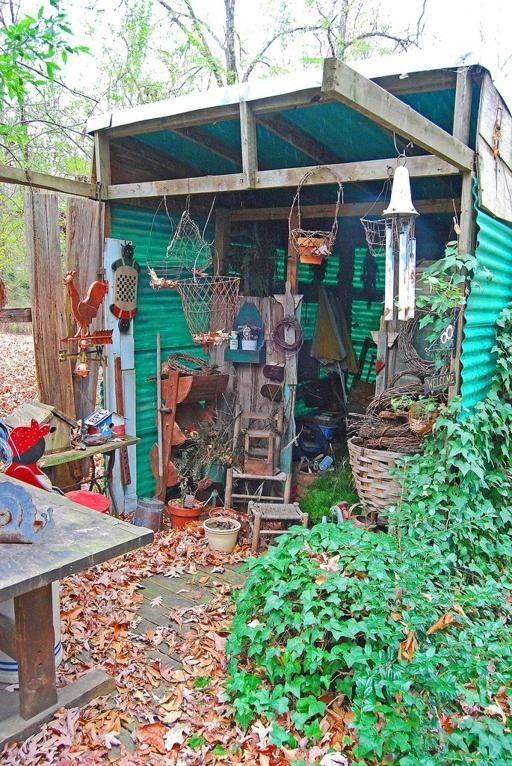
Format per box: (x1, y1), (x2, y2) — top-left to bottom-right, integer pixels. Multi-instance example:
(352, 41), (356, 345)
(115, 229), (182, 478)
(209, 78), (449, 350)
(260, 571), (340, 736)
(245, 501), (308, 551)
(64, 491), (110, 512)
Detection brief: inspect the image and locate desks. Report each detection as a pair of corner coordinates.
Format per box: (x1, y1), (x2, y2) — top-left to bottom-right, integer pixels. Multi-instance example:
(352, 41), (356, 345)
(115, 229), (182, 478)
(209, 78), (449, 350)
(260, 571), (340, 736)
(1, 473), (154, 752)
(38, 435), (141, 518)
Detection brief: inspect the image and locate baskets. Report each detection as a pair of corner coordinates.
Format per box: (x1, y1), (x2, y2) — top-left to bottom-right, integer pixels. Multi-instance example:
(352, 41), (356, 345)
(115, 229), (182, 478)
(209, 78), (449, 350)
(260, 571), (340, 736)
(288, 165), (340, 257)
(358, 215), (397, 258)
(174, 273), (242, 345)
(346, 435), (419, 524)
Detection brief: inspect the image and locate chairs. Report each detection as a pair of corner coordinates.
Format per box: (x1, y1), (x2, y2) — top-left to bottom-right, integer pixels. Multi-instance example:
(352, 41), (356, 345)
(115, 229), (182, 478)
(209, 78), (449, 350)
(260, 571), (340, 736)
(225, 404), (291, 510)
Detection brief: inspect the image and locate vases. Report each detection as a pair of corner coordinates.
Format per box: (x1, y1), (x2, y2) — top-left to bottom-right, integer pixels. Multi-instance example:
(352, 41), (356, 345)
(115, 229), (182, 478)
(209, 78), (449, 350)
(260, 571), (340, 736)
(298, 237), (324, 265)
(168, 499), (203, 530)
(204, 517), (242, 553)
